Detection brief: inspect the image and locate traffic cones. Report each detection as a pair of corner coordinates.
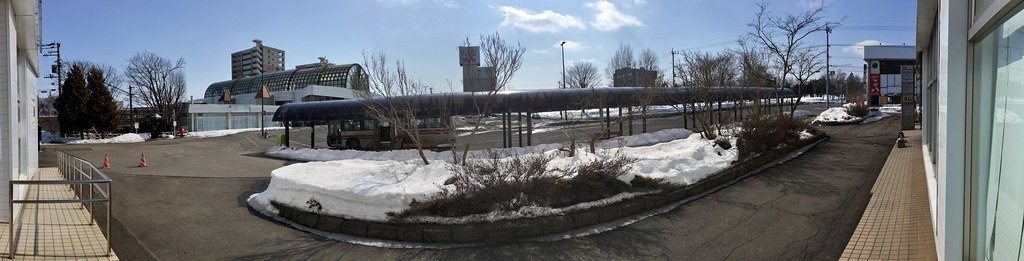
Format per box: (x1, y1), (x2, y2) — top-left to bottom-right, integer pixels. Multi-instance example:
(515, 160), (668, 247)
(98, 153), (111, 171)
(138, 151), (147, 168)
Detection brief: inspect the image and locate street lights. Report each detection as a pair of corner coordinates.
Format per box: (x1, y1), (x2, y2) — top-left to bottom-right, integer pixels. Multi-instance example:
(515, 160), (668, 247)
(252, 39), (264, 137)
(560, 40), (567, 121)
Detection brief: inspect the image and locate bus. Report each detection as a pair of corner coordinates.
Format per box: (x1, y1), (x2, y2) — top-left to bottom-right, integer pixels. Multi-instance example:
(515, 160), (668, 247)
(325, 112), (457, 151)
